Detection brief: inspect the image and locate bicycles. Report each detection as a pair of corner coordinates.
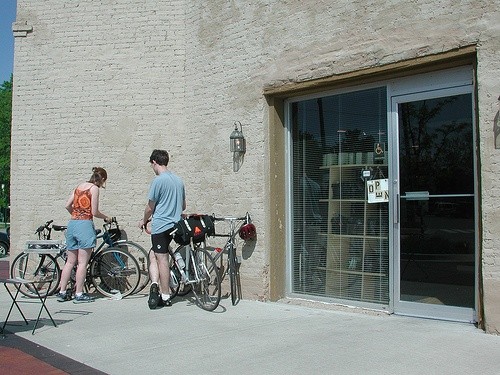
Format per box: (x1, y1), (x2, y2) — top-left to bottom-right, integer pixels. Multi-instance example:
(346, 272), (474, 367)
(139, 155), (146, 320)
(146, 213), (222, 312)
(11, 216), (140, 300)
(169, 212), (251, 305)
(19, 224), (150, 295)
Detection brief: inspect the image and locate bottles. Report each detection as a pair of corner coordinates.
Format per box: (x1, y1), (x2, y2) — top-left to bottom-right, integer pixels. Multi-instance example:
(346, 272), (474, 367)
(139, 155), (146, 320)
(175, 251), (185, 270)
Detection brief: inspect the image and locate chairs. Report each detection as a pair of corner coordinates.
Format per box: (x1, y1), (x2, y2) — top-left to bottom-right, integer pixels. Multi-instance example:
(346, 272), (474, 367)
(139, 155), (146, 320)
(0, 240), (62, 334)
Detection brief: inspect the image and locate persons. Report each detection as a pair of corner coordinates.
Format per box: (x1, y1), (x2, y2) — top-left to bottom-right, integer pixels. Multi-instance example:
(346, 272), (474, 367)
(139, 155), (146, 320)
(300, 167), (321, 290)
(56, 166), (112, 303)
(138, 151), (187, 309)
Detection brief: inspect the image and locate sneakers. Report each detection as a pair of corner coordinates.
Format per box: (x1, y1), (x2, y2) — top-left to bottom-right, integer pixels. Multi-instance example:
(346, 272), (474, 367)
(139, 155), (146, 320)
(57, 292), (73, 301)
(73, 293), (95, 303)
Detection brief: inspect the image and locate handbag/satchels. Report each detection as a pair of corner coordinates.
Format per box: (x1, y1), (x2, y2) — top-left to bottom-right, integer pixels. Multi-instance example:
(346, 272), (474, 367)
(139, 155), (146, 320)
(173, 214), (214, 245)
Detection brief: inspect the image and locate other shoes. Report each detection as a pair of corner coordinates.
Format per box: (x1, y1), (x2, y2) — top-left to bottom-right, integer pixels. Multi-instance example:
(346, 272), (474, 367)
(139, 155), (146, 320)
(148, 282), (159, 309)
(157, 295), (172, 306)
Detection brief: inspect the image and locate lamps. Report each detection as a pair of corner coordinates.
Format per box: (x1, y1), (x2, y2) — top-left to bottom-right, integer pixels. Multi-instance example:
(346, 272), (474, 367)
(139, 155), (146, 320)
(230, 121), (244, 152)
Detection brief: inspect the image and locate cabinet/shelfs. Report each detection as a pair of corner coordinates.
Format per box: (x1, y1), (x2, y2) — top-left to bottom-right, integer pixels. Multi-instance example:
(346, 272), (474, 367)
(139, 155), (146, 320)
(320, 164), (388, 299)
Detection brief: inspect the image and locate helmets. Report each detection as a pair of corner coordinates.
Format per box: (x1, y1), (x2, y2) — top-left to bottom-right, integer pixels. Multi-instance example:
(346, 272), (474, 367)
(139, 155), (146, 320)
(103, 228), (121, 245)
(239, 222), (256, 240)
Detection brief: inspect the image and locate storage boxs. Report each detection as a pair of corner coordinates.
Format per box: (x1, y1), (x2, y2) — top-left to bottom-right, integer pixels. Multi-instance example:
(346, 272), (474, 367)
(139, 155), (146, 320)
(323, 151), (388, 166)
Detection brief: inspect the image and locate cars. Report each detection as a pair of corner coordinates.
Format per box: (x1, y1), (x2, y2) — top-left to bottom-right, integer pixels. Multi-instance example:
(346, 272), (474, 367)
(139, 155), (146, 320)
(0, 231), (10, 257)
